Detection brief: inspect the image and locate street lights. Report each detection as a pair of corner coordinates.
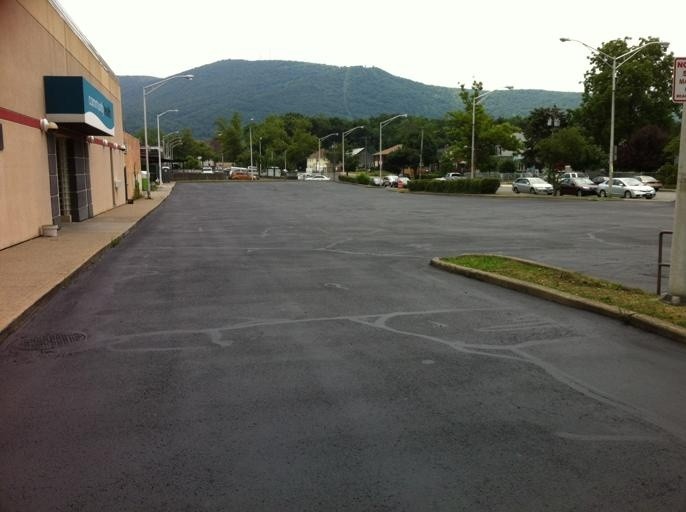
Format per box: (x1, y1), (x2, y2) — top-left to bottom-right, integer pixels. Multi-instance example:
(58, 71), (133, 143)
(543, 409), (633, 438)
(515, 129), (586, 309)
(471, 86), (513, 177)
(380, 114), (408, 179)
(249, 118), (262, 181)
(560, 39), (670, 176)
(318, 133), (338, 173)
(342, 126), (363, 175)
(143, 74), (194, 198)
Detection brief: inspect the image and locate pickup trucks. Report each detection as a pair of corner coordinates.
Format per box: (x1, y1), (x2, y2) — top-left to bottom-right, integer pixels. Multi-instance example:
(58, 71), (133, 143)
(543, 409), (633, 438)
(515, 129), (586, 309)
(446, 173), (467, 180)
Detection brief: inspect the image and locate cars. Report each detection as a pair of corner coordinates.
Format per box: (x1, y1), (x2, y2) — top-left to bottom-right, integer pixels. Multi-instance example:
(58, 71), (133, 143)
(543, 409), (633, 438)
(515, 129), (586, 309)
(635, 175), (662, 192)
(370, 176), (410, 188)
(512, 177), (553, 195)
(597, 178), (656, 199)
(223, 166), (259, 180)
(202, 166), (212, 173)
(297, 174), (330, 181)
(556, 172), (611, 196)
